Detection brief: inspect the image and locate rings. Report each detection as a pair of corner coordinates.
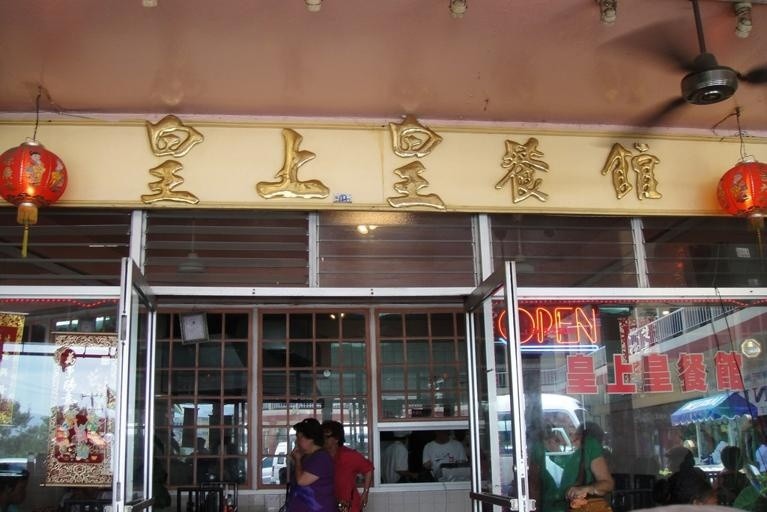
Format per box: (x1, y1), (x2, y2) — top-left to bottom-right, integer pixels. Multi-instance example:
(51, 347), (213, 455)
(363, 504), (366, 506)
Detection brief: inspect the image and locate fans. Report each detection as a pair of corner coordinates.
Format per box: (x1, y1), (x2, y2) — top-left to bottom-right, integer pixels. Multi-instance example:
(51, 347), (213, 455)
(600, 1), (766, 140)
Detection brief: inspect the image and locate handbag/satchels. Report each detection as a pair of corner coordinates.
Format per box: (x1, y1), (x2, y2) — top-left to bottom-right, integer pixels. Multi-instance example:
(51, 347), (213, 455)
(570, 496), (611, 512)
(334, 499), (351, 512)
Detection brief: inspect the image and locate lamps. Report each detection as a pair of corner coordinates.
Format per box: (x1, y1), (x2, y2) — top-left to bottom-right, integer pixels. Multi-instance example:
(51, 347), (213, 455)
(177, 219), (207, 273)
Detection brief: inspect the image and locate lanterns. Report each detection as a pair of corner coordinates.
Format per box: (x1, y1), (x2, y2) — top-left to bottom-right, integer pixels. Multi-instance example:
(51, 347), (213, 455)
(652, 221), (700, 280)
(714, 155), (767, 254)
(0, 135), (69, 259)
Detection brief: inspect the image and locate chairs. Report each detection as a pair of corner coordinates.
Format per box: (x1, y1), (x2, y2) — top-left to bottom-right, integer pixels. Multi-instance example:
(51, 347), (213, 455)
(177, 481), (237, 512)
(705, 471), (749, 507)
(609, 474), (659, 509)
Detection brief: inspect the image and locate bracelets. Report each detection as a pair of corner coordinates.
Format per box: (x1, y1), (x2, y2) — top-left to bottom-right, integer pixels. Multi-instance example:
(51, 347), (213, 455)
(364, 488), (370, 493)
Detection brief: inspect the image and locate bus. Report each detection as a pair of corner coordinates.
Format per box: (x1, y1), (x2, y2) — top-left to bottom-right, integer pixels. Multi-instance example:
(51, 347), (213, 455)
(497, 394), (592, 487)
(271, 417), (370, 483)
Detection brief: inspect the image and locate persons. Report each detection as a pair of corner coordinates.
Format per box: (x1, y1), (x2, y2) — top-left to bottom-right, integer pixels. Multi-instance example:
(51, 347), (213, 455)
(449, 429), (471, 461)
(319, 420), (374, 512)
(528, 400), (617, 512)
(283, 417), (338, 512)
(0, 462), (31, 512)
(560, 404), (766, 511)
(382, 430), (418, 483)
(50, 427), (273, 511)
(421, 430), (471, 483)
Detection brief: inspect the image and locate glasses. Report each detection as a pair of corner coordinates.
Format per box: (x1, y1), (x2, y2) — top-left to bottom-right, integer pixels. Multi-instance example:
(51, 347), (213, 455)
(325, 434), (333, 439)
